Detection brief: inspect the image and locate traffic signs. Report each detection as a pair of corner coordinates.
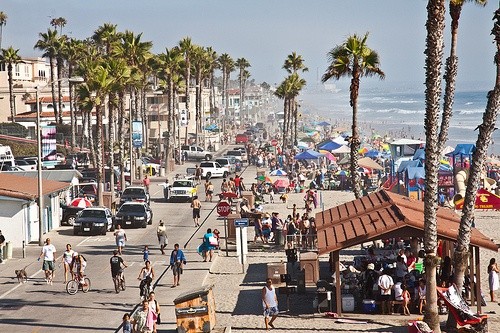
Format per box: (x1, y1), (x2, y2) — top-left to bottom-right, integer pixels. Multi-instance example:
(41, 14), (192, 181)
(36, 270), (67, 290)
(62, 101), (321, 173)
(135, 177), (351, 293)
(234, 218), (249, 227)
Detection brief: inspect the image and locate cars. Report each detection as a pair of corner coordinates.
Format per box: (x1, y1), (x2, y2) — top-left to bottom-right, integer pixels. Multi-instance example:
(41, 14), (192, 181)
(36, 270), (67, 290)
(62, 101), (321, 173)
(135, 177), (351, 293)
(119, 186), (150, 206)
(215, 122), (266, 173)
(199, 161), (229, 178)
(178, 145), (212, 161)
(115, 202), (152, 228)
(168, 180), (198, 202)
(60, 203), (85, 225)
(73, 208), (116, 236)
(0, 143), (160, 194)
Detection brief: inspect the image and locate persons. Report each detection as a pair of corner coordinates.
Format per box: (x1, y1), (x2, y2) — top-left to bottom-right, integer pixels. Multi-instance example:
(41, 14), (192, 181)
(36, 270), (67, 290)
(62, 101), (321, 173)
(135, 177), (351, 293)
(0, 104), (500, 333)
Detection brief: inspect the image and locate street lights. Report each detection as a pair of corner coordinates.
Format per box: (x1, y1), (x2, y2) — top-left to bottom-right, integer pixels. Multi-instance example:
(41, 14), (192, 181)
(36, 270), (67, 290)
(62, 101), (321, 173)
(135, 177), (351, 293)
(35, 75), (83, 247)
(173, 83), (200, 147)
(128, 91), (164, 186)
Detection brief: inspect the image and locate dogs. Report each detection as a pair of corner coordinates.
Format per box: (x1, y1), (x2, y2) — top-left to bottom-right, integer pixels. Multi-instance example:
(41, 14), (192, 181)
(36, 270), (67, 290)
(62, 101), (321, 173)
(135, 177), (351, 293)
(15, 269), (27, 283)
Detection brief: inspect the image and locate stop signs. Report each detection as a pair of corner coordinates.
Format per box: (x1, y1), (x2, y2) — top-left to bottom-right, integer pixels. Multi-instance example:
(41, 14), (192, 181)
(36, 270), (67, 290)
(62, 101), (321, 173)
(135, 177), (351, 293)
(218, 202), (230, 216)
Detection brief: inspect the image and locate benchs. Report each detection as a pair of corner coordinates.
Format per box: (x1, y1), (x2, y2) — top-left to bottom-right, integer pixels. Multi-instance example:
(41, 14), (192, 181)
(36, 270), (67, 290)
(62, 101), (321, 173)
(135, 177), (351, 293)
(376, 299), (450, 316)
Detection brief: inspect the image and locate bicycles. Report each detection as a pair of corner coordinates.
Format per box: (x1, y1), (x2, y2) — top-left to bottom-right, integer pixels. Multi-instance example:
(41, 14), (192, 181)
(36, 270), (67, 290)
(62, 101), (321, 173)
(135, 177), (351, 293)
(66, 271), (90, 296)
(137, 277), (154, 302)
(113, 266), (127, 294)
